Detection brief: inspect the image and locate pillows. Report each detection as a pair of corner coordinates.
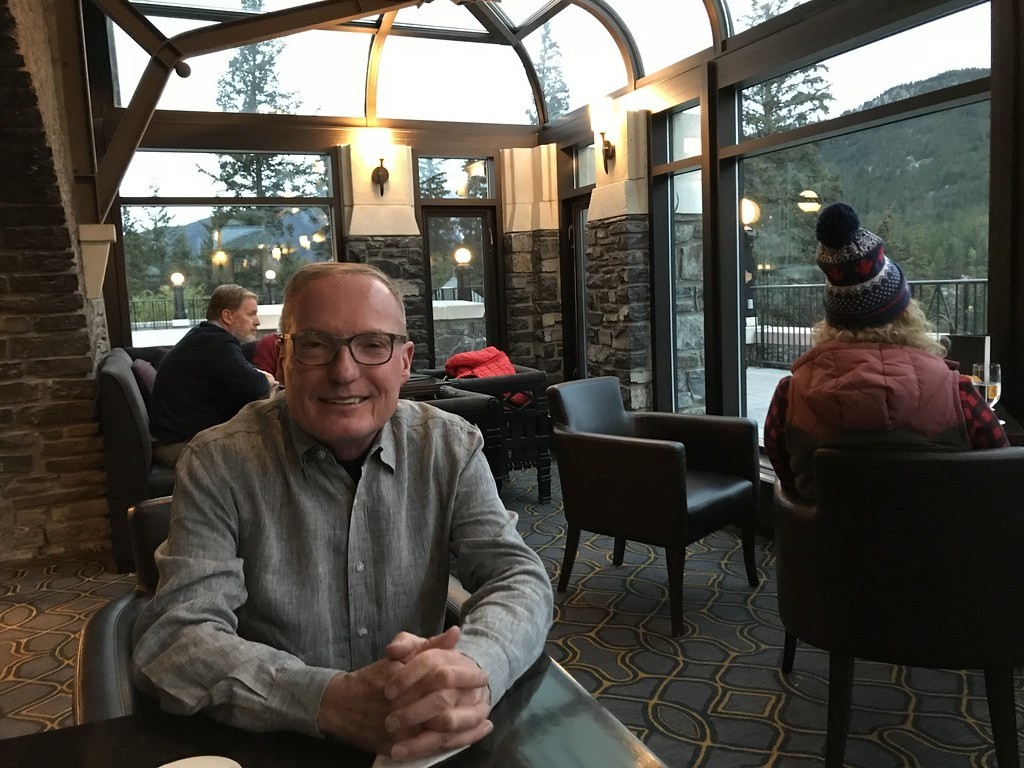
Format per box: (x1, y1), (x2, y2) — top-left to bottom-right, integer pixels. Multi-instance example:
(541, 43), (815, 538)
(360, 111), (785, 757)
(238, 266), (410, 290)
(130, 358), (158, 395)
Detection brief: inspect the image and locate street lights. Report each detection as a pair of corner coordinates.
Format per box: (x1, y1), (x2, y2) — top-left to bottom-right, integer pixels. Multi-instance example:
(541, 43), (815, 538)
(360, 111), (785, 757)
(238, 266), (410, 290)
(740, 197), (759, 326)
(170, 270), (186, 319)
(264, 269), (277, 304)
(455, 246), (472, 299)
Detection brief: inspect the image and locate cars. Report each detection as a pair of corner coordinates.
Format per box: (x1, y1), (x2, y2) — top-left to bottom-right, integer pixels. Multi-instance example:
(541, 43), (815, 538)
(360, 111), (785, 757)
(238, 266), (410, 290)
(747, 265), (826, 330)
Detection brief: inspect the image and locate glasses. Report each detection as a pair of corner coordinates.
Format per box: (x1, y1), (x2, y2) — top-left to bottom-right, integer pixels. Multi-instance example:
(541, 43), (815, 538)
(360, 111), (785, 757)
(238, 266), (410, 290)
(281, 331), (406, 366)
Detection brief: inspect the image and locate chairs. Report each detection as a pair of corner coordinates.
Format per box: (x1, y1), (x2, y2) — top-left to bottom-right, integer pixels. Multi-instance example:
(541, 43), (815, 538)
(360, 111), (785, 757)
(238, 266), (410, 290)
(542, 375), (760, 637)
(72, 494), (468, 726)
(97, 342), (553, 574)
(771, 446), (1024, 767)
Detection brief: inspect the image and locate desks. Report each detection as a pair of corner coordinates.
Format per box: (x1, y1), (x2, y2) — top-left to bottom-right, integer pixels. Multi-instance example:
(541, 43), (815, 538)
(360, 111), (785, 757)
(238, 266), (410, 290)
(993, 402), (1024, 446)
(399, 371), (450, 401)
(1, 650), (669, 767)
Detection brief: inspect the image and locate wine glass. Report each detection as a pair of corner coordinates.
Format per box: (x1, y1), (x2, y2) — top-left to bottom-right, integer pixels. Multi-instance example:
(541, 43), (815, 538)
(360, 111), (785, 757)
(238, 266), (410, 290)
(972, 363), (1006, 426)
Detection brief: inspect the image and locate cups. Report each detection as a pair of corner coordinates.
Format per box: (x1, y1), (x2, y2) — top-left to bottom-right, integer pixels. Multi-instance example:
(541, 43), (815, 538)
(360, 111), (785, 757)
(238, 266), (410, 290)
(157, 755), (242, 768)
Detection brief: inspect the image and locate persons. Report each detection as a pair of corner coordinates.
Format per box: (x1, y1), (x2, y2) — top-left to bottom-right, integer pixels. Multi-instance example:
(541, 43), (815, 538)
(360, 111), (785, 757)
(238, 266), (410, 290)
(764, 199), (1009, 500)
(132, 261), (555, 759)
(149, 284), (279, 470)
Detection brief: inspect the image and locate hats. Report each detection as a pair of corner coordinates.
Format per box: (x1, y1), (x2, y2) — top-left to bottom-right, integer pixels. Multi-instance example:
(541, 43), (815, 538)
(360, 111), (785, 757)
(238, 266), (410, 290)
(817, 202), (913, 326)
